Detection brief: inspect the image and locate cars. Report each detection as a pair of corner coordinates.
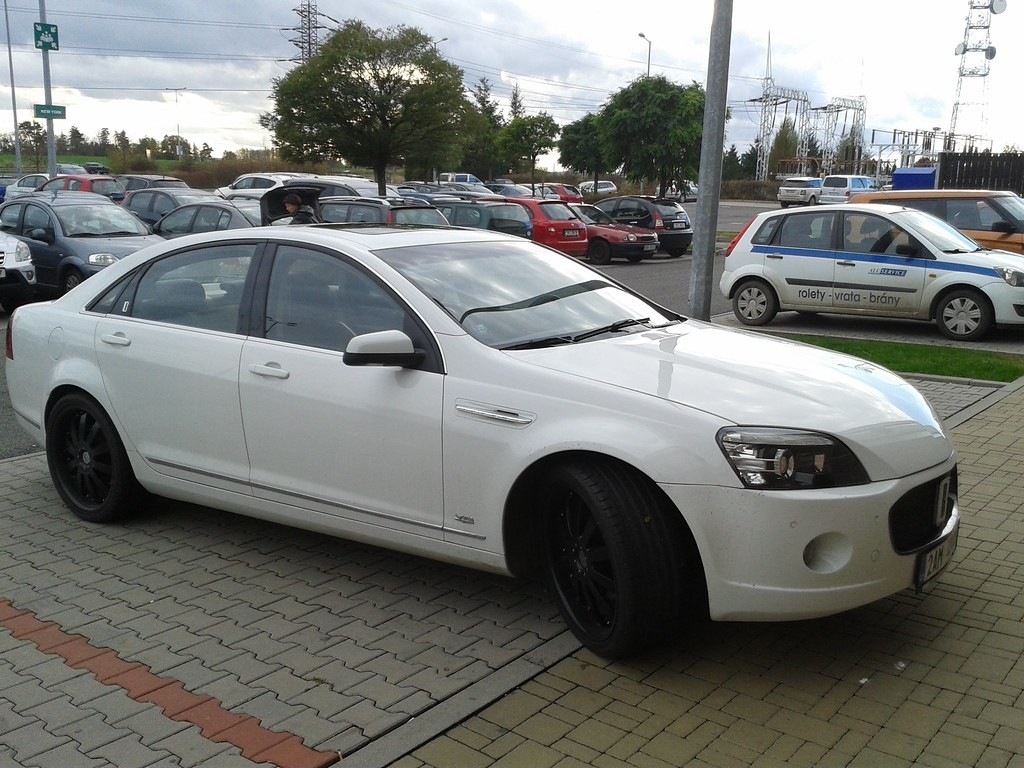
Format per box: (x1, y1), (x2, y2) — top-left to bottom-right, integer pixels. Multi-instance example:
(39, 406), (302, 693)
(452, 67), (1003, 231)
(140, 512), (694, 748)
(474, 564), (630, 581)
(833, 190), (1024, 255)
(777, 177), (823, 208)
(955, 201), (1006, 227)
(82, 162), (111, 175)
(152, 184), (329, 239)
(574, 181), (618, 197)
(4, 223), (962, 669)
(287, 176), (403, 200)
(566, 203), (661, 265)
(719, 205), (1023, 342)
(389, 173), (659, 265)
(593, 195), (694, 259)
(656, 180), (698, 203)
(0, 173), (224, 306)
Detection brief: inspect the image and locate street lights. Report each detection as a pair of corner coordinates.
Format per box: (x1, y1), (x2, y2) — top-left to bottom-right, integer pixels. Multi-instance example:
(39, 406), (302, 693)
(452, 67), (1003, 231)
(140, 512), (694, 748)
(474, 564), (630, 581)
(434, 38), (448, 56)
(638, 32), (652, 77)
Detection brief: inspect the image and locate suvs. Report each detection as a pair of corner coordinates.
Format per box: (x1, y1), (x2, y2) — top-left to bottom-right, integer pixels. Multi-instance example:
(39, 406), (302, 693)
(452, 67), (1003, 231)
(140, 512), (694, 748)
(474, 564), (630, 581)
(318, 195), (451, 226)
(215, 172), (301, 200)
(56, 163), (88, 175)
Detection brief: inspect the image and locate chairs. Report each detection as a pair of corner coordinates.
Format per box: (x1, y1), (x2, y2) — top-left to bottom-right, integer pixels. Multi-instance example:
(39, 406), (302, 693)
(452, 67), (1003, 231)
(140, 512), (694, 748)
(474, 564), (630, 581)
(275, 264), (355, 352)
(791, 219), (816, 246)
(153, 275), (224, 324)
(30, 210), (48, 227)
(859, 218), (882, 251)
(202, 208), (225, 231)
(844, 221), (855, 247)
(74, 205), (100, 233)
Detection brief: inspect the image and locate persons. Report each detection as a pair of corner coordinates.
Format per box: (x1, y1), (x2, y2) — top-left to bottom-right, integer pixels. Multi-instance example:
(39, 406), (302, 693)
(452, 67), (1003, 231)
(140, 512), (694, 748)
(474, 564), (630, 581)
(285, 194), (324, 225)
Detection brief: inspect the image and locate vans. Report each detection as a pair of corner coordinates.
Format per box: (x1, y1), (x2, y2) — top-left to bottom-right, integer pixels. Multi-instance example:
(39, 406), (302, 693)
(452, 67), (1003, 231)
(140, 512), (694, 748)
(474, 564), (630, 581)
(819, 176), (882, 205)
